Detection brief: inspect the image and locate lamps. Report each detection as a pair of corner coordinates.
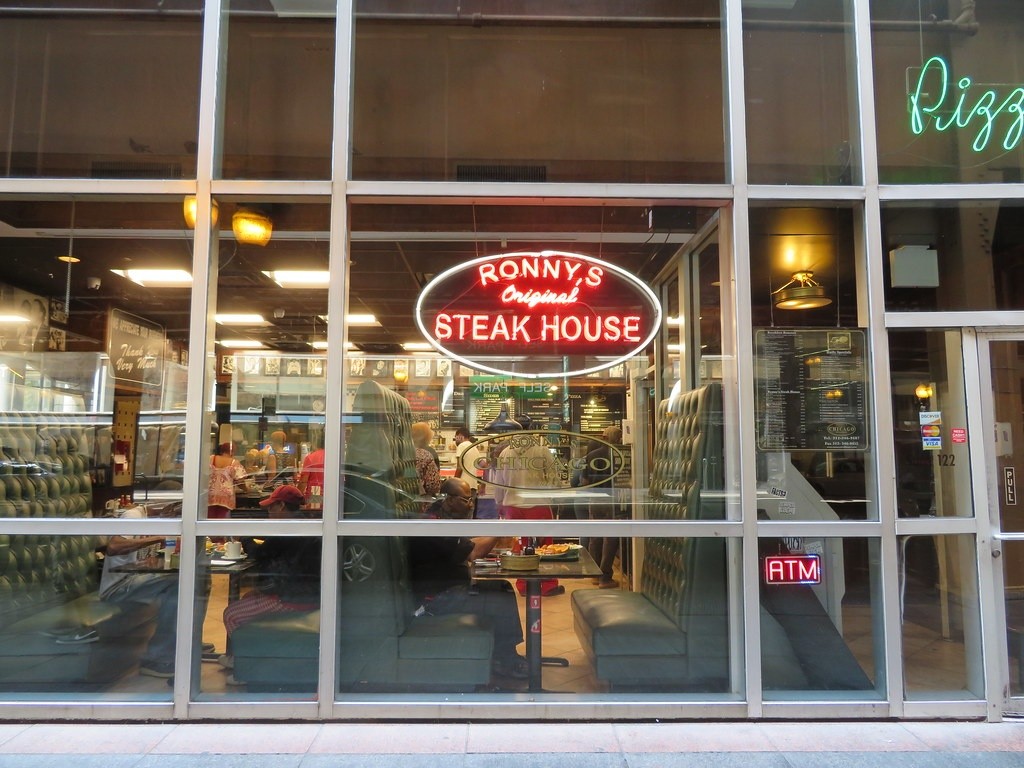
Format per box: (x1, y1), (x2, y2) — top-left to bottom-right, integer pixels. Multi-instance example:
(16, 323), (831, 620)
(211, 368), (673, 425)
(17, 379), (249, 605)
(184, 194), (219, 228)
(232, 203), (273, 247)
(771, 271), (832, 309)
(916, 382), (932, 410)
(483, 370), (522, 431)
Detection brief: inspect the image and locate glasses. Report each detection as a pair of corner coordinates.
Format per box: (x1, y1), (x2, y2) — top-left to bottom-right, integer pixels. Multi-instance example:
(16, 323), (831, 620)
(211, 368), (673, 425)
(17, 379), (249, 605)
(455, 496), (472, 502)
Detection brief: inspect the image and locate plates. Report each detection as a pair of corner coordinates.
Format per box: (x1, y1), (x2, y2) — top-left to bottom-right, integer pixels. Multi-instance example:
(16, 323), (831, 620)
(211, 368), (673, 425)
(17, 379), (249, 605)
(221, 555), (247, 560)
(476, 562), (500, 567)
(215, 548), (243, 555)
(539, 552), (567, 557)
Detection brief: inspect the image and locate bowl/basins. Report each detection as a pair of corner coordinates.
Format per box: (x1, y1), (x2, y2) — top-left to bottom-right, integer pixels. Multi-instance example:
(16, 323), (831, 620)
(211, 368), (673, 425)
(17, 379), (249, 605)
(155, 547), (165, 556)
(569, 545), (583, 554)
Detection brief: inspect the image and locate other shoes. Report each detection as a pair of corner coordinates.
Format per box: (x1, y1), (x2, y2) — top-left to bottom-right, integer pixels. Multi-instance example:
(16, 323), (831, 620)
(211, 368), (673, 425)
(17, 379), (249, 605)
(598, 579), (619, 589)
(201, 642), (214, 654)
(139, 660), (175, 677)
(468, 583), (478, 594)
(502, 581), (515, 592)
(592, 577), (598, 584)
(501, 654), (531, 678)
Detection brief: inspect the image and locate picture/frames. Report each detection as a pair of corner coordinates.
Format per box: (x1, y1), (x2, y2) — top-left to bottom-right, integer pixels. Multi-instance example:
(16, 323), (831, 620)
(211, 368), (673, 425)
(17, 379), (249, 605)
(171, 346), (189, 372)
(215, 354), (487, 378)
(672, 359), (723, 379)
(0, 276), (70, 352)
(585, 362), (626, 379)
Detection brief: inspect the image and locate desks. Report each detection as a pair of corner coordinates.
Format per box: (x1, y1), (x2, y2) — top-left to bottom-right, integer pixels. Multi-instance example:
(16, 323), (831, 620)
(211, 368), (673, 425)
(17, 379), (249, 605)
(109, 543), (263, 687)
(473, 544), (604, 692)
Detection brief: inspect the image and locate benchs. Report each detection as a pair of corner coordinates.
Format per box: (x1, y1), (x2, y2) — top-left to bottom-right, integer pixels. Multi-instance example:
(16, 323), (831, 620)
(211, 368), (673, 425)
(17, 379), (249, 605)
(231, 378), (495, 692)
(0, 381), (159, 693)
(571, 383), (729, 692)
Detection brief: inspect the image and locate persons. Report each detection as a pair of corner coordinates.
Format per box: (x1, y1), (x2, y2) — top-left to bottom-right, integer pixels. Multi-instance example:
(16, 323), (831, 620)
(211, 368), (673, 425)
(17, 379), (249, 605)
(208, 441), (249, 543)
(289, 359), (300, 375)
(570, 440), (603, 549)
(310, 359), (321, 373)
(439, 361), (449, 376)
(50, 329), (63, 350)
(351, 360), (366, 375)
(583, 426), (623, 588)
(455, 428), (489, 519)
(224, 358), (233, 370)
(298, 430), (325, 516)
(52, 302), (64, 321)
(99, 479), (215, 677)
(217, 485), (321, 684)
(407, 477), (529, 680)
(264, 431), (297, 483)
(411, 422), (440, 496)
(373, 361), (385, 376)
(491, 418), (565, 597)
(17, 297), (49, 346)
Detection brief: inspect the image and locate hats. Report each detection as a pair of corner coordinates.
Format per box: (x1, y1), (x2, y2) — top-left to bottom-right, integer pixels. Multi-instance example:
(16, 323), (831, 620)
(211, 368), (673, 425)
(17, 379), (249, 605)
(603, 426), (623, 443)
(259, 485), (304, 506)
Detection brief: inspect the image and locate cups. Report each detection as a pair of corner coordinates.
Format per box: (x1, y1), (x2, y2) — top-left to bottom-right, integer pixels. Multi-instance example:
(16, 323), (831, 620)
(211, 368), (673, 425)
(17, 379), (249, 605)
(244, 478), (254, 492)
(300, 444), (307, 466)
(224, 542), (241, 557)
(170, 553), (180, 569)
(105, 500), (115, 509)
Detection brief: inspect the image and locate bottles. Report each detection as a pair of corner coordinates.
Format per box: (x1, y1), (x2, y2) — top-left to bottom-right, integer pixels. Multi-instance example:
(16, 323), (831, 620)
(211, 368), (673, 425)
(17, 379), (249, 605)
(511, 536), (524, 556)
(525, 537), (535, 555)
(165, 536), (176, 561)
(115, 495), (132, 517)
(410, 413), (446, 450)
(206, 536), (212, 550)
